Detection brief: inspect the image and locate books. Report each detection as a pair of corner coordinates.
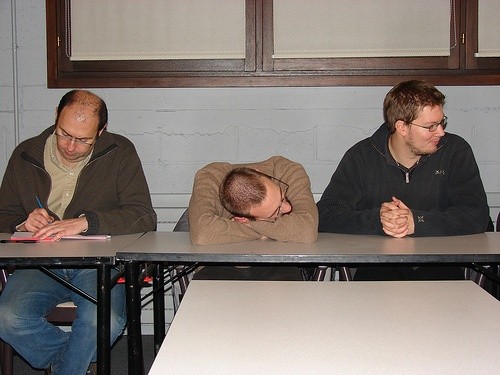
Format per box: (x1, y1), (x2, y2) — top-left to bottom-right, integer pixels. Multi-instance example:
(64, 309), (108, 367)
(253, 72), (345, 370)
(11, 231), (52, 240)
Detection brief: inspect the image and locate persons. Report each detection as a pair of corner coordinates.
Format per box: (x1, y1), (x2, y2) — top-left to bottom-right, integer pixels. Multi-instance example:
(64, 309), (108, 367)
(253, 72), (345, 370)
(0, 90), (157, 375)
(173, 156), (319, 281)
(317, 80), (490, 281)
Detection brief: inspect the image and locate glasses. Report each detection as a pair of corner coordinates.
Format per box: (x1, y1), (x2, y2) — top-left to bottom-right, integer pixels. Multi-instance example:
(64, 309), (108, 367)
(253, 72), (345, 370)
(54, 132), (97, 146)
(406, 115), (448, 133)
(256, 173), (290, 223)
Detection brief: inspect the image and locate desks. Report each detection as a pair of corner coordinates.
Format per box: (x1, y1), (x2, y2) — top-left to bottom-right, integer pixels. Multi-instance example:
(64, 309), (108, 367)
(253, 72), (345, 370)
(0, 231), (500, 375)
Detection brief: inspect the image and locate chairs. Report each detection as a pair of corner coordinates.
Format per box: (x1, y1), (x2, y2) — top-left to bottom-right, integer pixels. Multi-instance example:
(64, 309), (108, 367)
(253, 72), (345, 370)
(340, 217), (495, 287)
(0, 268), (79, 375)
(171, 210), (328, 295)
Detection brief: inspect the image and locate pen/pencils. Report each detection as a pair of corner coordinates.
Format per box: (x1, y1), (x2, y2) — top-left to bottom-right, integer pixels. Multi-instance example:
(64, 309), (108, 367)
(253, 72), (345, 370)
(35, 196), (52, 224)
(0, 239), (40, 244)
